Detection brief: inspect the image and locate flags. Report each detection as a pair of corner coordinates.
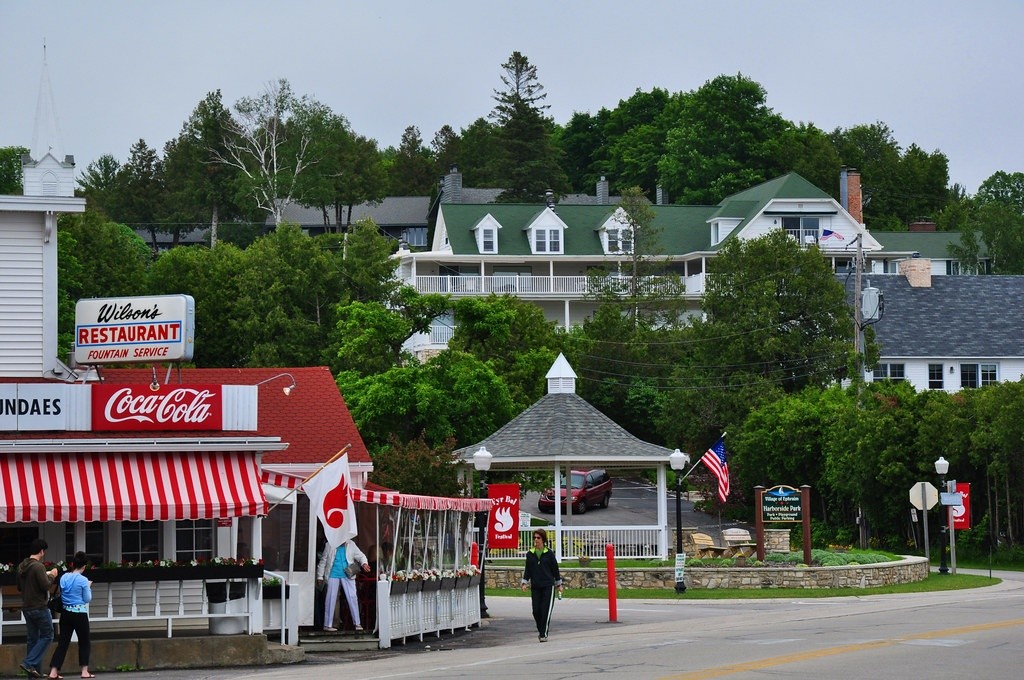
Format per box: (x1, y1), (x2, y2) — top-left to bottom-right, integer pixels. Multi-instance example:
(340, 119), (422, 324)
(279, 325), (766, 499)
(303, 452), (358, 548)
(701, 437), (730, 502)
(821, 229), (846, 241)
(488, 483), (519, 550)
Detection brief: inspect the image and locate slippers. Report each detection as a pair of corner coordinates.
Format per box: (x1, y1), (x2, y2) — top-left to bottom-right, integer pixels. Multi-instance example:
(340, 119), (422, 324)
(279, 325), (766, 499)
(48, 674), (64, 680)
(81, 673), (96, 678)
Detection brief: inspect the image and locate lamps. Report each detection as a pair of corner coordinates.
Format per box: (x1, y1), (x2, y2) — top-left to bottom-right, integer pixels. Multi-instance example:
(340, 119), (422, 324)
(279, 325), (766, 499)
(255, 373), (297, 396)
(149, 366), (160, 391)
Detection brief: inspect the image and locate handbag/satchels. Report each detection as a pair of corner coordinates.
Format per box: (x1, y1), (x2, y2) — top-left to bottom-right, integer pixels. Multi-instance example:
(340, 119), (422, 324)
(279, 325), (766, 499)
(343, 562), (361, 578)
(46, 570), (65, 613)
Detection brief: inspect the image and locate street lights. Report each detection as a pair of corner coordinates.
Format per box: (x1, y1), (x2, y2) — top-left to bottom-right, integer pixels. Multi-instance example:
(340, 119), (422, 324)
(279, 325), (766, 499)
(935, 456), (951, 574)
(668, 449), (686, 593)
(472, 446), (493, 618)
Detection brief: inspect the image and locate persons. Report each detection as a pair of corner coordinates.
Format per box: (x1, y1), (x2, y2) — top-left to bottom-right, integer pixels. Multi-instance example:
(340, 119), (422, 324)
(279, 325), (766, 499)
(48, 551), (96, 680)
(317, 540), (371, 630)
(16, 539), (58, 680)
(520, 529), (563, 641)
(361, 542), (434, 620)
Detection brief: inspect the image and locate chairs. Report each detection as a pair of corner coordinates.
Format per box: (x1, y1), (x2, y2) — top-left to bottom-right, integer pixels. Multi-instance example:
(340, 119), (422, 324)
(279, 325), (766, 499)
(689, 533), (728, 559)
(722, 528), (758, 561)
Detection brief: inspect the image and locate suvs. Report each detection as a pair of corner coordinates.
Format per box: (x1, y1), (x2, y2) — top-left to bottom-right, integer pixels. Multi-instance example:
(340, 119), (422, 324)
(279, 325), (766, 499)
(538, 467), (612, 514)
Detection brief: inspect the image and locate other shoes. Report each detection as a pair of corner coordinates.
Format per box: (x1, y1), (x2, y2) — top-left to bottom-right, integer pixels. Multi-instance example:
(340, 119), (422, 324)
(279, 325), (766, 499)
(19, 662), (41, 678)
(355, 625), (363, 630)
(323, 626), (338, 632)
(539, 637), (548, 642)
(28, 673), (48, 680)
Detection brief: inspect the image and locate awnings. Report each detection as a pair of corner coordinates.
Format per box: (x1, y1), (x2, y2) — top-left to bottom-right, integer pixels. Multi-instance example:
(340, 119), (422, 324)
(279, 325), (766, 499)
(1, 450), (268, 522)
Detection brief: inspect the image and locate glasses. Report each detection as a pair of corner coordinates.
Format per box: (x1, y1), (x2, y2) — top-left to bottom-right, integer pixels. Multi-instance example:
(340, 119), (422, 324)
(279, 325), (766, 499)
(533, 537), (541, 539)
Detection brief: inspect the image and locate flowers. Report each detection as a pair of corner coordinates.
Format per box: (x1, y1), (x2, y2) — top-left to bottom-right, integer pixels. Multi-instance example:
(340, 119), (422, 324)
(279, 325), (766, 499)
(0, 556), (265, 575)
(392, 565), (482, 581)
